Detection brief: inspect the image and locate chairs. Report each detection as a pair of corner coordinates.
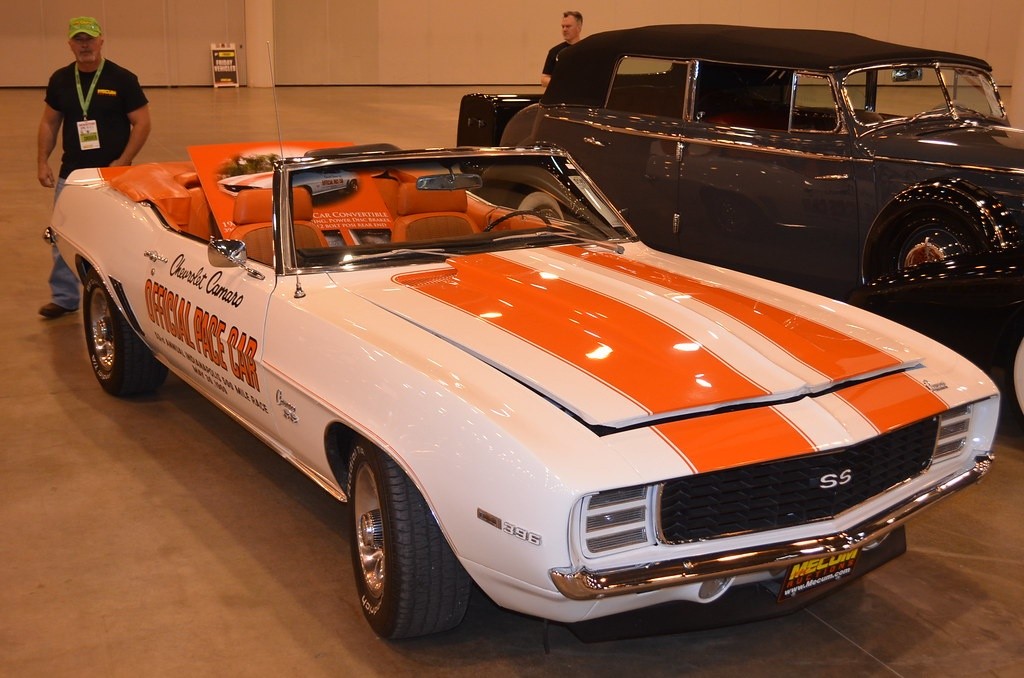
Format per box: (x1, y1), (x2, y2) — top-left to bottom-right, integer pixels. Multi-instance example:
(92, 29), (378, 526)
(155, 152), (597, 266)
(234, 186), (331, 266)
(706, 111), (791, 133)
(387, 183), (481, 243)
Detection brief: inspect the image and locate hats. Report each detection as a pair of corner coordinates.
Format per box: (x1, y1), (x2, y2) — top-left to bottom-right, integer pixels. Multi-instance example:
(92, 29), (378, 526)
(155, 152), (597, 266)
(68, 16), (103, 38)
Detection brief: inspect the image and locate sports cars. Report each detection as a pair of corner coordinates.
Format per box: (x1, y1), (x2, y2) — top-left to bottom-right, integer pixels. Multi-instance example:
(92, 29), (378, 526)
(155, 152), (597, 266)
(40, 143), (1004, 642)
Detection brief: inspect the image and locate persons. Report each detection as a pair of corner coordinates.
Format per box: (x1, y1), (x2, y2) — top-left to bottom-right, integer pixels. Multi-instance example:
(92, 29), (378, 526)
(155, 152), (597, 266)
(38, 17), (150, 319)
(542, 11), (582, 87)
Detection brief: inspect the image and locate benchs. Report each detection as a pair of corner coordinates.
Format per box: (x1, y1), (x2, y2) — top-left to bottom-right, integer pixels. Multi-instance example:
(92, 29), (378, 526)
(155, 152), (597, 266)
(607, 81), (741, 113)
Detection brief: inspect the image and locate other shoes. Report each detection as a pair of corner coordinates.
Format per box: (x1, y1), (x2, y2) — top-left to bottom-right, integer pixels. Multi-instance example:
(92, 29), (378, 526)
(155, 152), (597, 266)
(39, 303), (77, 318)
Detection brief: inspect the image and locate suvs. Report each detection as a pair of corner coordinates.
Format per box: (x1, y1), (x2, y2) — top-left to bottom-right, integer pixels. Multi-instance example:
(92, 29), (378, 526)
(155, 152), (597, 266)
(453, 22), (1023, 428)
